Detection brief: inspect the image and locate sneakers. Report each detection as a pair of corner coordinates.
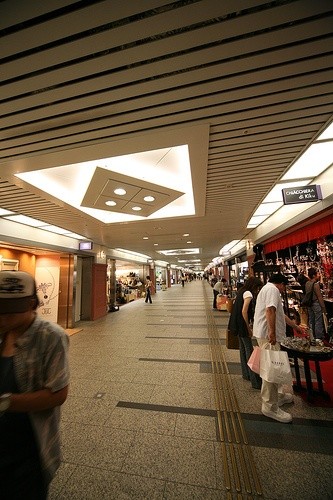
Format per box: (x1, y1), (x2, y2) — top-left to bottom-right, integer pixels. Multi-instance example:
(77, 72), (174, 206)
(278, 392), (293, 406)
(260, 401), (292, 424)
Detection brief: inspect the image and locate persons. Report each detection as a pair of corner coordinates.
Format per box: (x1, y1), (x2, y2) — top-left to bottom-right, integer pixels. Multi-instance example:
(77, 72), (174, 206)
(0, 275), (70, 500)
(253, 273), (295, 424)
(305, 268), (329, 347)
(227, 275), (262, 392)
(213, 277), (227, 309)
(181, 272), (203, 287)
(145, 276), (153, 304)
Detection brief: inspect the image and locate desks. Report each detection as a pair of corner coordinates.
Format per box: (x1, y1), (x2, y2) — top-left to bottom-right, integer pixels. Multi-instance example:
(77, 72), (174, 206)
(281, 338), (333, 407)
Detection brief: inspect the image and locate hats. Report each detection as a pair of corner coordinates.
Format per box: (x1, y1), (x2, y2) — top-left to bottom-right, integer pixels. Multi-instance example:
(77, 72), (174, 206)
(0, 269), (37, 315)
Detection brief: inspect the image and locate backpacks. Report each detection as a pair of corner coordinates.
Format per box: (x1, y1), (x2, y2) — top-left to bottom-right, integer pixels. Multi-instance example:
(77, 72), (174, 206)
(298, 281), (316, 308)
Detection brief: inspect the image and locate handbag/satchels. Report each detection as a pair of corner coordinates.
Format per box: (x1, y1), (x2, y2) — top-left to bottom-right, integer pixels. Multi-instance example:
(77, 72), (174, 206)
(246, 346), (261, 374)
(260, 342), (293, 384)
